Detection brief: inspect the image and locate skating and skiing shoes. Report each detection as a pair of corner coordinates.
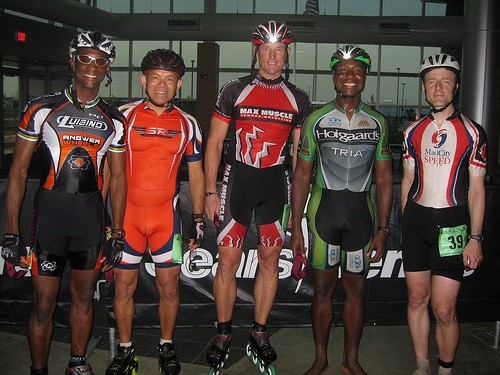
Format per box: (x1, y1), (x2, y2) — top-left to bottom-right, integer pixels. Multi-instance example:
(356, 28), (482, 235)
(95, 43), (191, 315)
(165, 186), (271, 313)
(246, 321), (277, 375)
(65, 362), (95, 375)
(206, 319), (233, 375)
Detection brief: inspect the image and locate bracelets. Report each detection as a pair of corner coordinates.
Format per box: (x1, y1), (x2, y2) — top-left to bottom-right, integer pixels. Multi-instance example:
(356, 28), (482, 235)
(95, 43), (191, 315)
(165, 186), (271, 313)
(206, 192), (213, 196)
(191, 214), (207, 219)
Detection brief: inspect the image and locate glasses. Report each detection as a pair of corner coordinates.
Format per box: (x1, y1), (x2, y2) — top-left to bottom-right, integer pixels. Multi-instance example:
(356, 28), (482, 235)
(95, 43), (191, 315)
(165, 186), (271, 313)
(76, 54), (109, 66)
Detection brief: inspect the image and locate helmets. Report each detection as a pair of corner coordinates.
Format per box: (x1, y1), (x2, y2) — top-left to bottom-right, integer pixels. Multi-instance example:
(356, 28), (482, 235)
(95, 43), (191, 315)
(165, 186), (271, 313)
(419, 53), (461, 75)
(252, 21), (294, 44)
(330, 45), (371, 69)
(141, 48), (185, 75)
(69, 29), (116, 62)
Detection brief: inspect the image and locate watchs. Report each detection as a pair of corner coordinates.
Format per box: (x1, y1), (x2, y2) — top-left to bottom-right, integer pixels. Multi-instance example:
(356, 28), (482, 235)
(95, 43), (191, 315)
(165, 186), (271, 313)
(378, 226), (391, 235)
(111, 229), (125, 238)
(468, 234), (484, 242)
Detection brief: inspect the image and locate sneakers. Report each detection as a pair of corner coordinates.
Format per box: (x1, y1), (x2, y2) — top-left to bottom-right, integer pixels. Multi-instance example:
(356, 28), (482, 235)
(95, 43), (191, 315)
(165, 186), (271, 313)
(105, 344), (140, 375)
(156, 342), (181, 375)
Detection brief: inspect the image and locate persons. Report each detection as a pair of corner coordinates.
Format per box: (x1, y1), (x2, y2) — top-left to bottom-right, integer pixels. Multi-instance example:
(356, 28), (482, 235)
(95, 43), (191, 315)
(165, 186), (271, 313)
(0, 31), (126, 375)
(101, 48), (207, 375)
(291, 45), (393, 375)
(402, 54), (487, 374)
(202, 19), (314, 374)
